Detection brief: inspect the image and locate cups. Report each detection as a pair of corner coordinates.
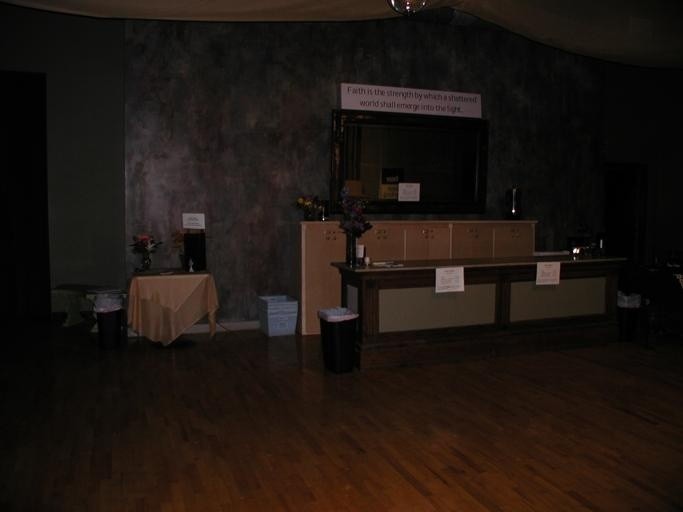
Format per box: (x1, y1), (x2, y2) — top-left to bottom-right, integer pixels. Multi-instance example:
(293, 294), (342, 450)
(365, 257), (372, 270)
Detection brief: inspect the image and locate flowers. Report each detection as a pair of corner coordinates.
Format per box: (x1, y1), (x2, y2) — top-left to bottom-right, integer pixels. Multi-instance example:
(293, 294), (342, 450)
(338, 194), (373, 235)
(131, 233), (162, 252)
(171, 229), (186, 252)
(298, 195), (326, 213)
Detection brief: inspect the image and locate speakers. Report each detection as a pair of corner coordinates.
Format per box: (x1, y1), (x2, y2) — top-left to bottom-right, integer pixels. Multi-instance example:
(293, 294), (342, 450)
(183, 233), (206, 271)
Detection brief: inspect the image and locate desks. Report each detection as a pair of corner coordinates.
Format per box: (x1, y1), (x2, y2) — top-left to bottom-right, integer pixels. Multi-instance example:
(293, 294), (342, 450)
(130, 273), (213, 345)
(331, 252), (622, 353)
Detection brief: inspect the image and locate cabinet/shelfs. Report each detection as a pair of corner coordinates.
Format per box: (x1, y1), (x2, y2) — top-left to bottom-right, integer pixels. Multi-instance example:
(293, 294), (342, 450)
(300, 218), (539, 337)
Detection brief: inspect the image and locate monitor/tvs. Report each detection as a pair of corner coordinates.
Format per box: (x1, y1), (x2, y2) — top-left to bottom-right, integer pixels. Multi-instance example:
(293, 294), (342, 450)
(344, 119), (487, 217)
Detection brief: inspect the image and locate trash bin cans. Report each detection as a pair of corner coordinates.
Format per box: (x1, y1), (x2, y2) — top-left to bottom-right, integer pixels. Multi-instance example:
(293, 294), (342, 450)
(617, 291), (651, 342)
(95, 307), (124, 346)
(317, 306), (359, 374)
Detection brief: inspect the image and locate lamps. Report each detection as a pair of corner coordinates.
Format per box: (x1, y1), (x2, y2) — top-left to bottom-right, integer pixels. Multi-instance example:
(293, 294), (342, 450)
(392, 0), (426, 14)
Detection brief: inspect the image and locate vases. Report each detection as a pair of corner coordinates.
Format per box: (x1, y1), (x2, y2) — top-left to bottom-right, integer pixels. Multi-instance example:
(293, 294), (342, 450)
(344, 233), (357, 266)
(180, 253), (189, 269)
(142, 254), (151, 269)
(306, 212), (312, 218)
